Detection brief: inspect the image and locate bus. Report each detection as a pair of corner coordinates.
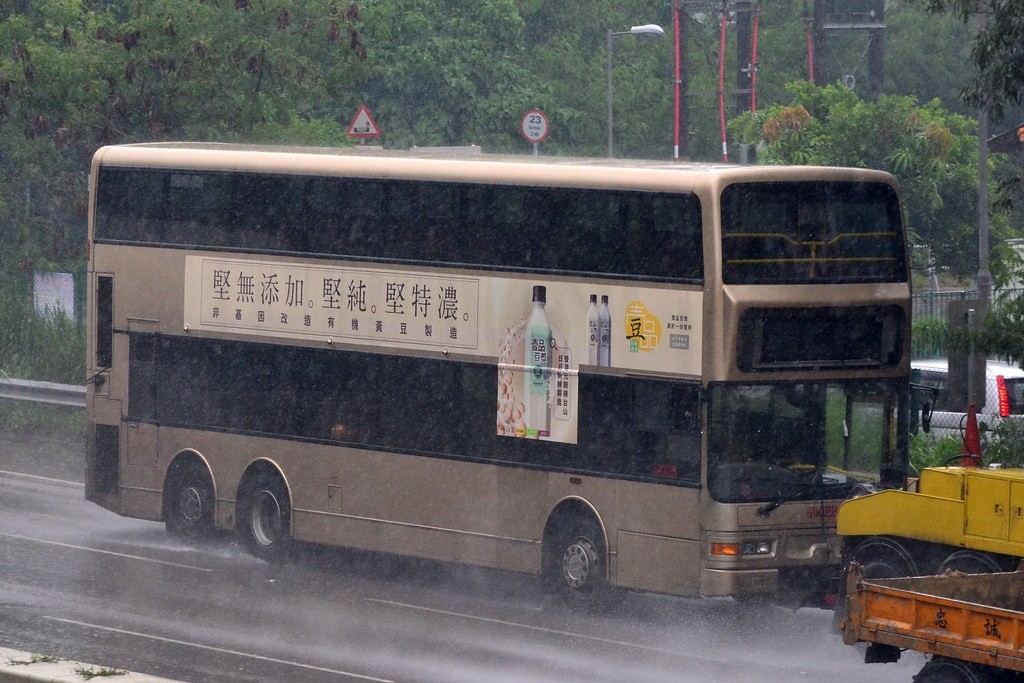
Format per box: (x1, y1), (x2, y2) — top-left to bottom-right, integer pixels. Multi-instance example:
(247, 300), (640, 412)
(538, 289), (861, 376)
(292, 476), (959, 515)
(81, 141), (914, 618)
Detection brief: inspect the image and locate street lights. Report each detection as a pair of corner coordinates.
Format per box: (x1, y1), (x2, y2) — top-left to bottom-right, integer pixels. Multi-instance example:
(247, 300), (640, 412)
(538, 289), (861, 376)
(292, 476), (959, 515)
(606, 24), (666, 156)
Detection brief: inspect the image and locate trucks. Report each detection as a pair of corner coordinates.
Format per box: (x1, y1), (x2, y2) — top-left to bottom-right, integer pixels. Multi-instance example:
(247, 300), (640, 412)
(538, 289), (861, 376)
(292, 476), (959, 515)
(831, 557), (1024, 683)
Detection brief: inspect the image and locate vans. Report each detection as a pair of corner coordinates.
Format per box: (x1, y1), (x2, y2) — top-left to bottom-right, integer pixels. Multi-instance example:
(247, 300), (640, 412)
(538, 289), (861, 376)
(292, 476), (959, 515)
(906, 356), (1024, 442)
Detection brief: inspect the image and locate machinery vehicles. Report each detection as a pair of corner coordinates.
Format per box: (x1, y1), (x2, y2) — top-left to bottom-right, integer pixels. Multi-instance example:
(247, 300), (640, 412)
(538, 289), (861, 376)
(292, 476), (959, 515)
(838, 464), (1024, 611)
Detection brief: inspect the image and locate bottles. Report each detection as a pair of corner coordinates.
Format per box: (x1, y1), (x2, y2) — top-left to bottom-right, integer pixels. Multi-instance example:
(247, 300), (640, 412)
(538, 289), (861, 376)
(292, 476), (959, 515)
(587, 295), (600, 366)
(523, 286), (553, 439)
(600, 295), (611, 366)
